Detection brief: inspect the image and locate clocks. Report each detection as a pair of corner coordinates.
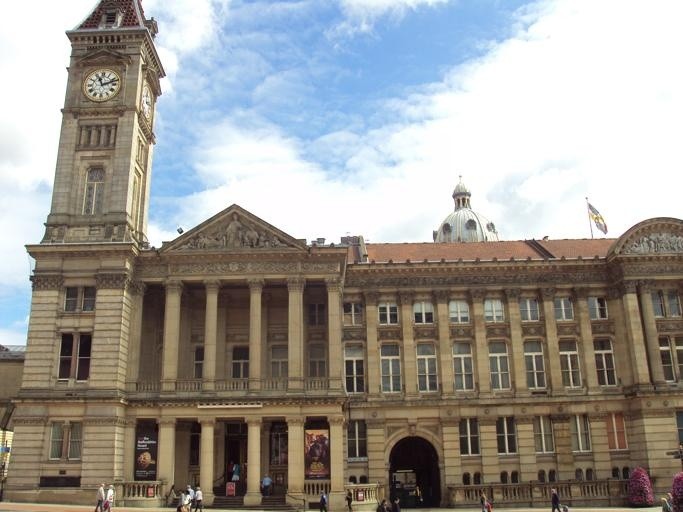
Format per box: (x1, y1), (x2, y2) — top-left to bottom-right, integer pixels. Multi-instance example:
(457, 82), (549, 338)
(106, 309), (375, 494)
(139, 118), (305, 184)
(142, 85), (154, 120)
(83, 68), (121, 102)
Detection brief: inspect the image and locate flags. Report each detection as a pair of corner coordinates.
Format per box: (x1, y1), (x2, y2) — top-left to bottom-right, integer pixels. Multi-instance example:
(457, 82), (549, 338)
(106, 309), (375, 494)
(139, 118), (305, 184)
(588, 202), (608, 235)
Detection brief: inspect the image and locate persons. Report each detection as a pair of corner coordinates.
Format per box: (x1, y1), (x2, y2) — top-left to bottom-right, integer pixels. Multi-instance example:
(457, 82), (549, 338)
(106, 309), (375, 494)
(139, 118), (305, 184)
(551, 489), (562, 512)
(103, 484), (114, 512)
(94, 483), (105, 512)
(414, 486), (424, 505)
(174, 210), (290, 249)
(660, 497), (673, 512)
(167, 483), (203, 512)
(664, 492), (673, 507)
(260, 473), (273, 498)
(380, 499), (388, 511)
(345, 488), (353, 512)
(390, 496), (401, 512)
(479, 491), (492, 512)
(319, 490), (328, 512)
(231, 463), (240, 484)
(625, 230), (683, 254)
